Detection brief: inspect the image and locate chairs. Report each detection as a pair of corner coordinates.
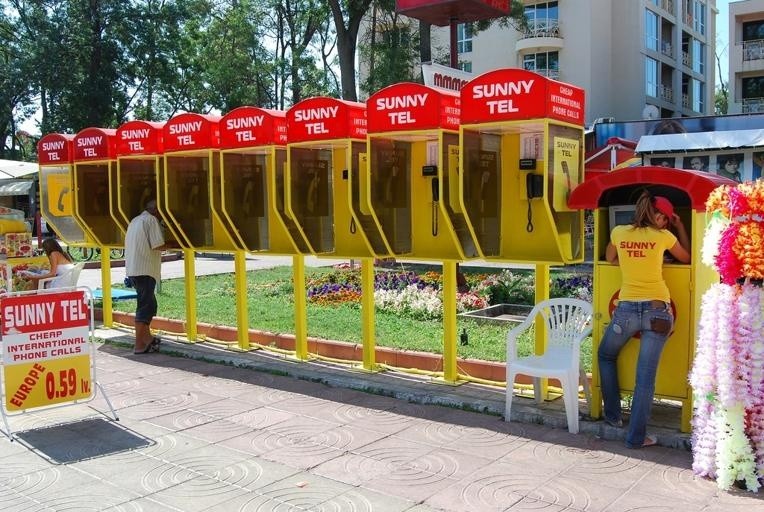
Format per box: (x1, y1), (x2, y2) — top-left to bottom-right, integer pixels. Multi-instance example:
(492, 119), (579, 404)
(505, 298), (593, 435)
(51, 262), (85, 292)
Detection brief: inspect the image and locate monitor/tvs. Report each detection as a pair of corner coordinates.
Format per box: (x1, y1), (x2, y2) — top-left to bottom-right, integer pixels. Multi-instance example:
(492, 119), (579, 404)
(608, 204), (637, 232)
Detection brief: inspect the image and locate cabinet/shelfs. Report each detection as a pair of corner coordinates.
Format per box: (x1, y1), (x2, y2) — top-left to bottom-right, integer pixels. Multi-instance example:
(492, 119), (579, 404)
(597, 264), (720, 399)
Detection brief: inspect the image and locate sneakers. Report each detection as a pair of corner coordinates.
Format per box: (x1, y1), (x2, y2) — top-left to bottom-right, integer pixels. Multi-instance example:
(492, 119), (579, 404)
(641, 435), (657, 447)
(606, 416), (623, 427)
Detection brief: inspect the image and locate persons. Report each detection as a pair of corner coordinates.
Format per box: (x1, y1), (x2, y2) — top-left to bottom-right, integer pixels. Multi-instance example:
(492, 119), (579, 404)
(125, 200), (168, 354)
(598, 191), (691, 448)
(26, 239), (73, 289)
(689, 156), (708, 172)
(717, 154), (742, 183)
(661, 158), (674, 168)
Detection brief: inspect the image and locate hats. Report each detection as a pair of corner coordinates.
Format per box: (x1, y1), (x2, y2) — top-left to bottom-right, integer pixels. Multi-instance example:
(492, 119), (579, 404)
(653, 197), (674, 228)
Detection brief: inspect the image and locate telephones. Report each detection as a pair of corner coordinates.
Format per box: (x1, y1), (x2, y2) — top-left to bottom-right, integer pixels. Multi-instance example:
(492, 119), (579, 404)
(526, 173), (544, 199)
(432, 177), (439, 201)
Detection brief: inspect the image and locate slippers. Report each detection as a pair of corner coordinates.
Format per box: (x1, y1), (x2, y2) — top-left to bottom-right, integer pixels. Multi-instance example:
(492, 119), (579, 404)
(134, 336), (160, 354)
